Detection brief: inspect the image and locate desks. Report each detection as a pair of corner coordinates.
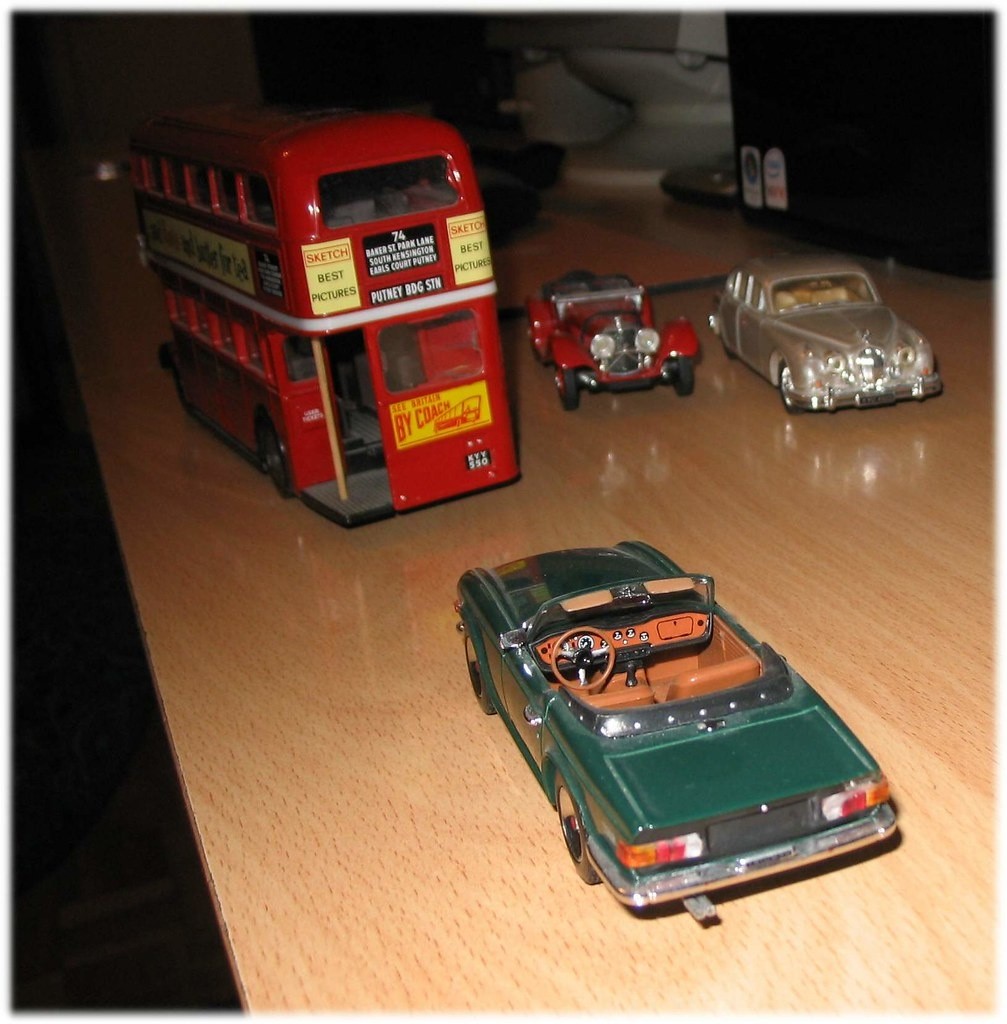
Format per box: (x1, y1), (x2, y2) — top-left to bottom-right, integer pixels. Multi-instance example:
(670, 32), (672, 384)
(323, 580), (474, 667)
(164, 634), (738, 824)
(17, 14), (995, 1011)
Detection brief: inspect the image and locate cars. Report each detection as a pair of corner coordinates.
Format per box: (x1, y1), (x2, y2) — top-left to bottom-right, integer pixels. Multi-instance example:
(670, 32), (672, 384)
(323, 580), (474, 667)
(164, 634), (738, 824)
(453, 538), (902, 922)
(712, 254), (942, 414)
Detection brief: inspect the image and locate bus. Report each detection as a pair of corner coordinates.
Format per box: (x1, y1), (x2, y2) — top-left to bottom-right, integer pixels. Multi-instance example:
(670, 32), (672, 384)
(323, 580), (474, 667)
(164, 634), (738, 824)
(107, 110), (527, 523)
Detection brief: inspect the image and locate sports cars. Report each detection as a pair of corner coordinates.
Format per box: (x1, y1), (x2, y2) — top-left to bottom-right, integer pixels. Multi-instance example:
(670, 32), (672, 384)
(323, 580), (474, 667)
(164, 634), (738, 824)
(524, 270), (700, 411)
